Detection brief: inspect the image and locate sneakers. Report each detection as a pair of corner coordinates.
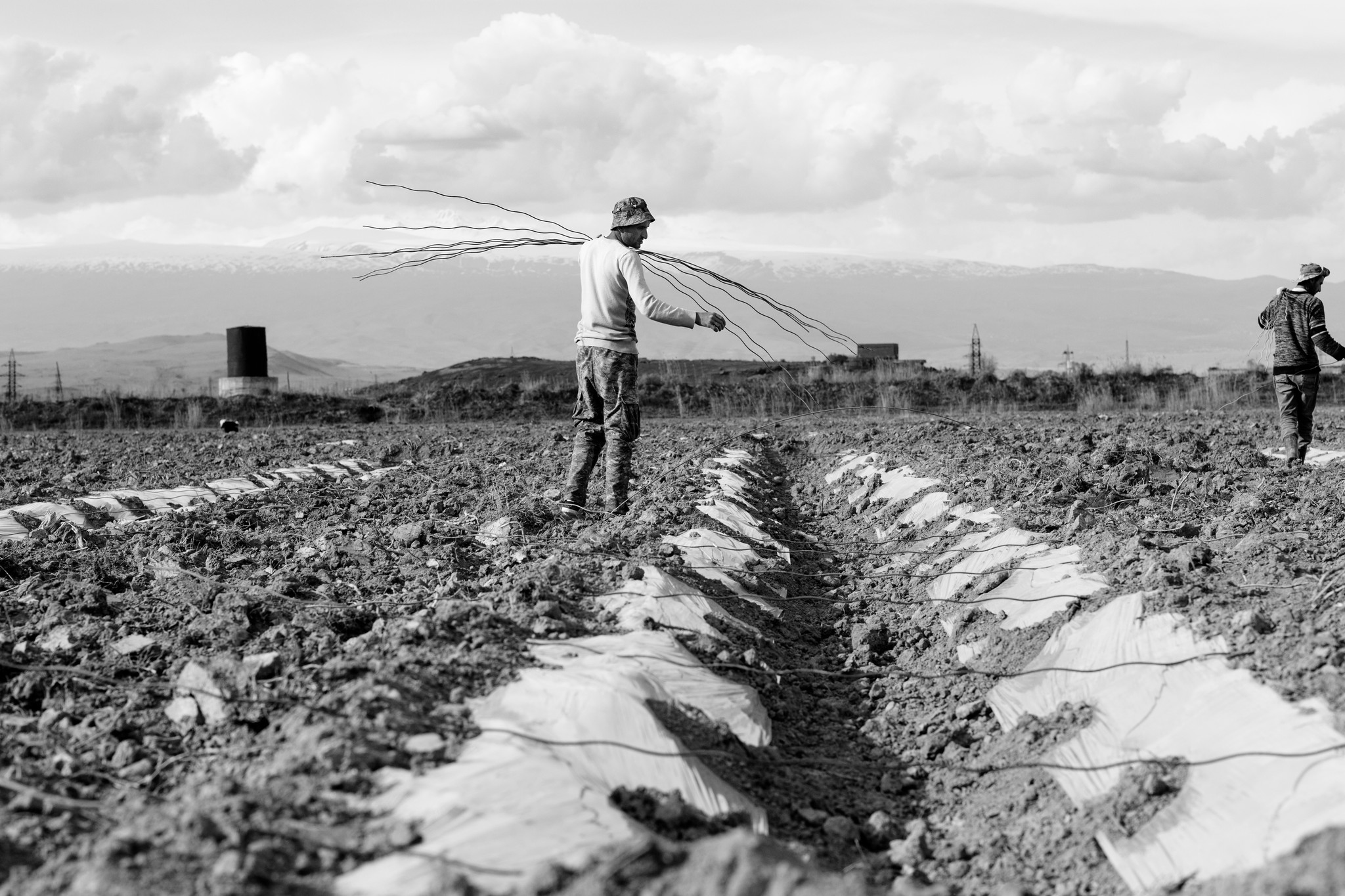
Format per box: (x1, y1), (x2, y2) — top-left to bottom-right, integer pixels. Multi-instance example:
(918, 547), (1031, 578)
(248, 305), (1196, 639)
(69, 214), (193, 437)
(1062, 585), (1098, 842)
(562, 501), (585, 519)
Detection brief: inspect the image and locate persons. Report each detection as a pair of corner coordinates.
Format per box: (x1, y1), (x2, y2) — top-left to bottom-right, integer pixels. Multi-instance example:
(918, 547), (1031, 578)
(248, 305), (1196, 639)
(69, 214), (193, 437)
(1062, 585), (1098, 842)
(1257, 262), (1345, 467)
(561, 198), (726, 522)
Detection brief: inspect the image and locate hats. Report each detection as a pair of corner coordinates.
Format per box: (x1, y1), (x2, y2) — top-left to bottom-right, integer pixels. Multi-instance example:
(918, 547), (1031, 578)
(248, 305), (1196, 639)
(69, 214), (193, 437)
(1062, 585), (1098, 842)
(609, 197), (655, 231)
(1290, 263), (1330, 283)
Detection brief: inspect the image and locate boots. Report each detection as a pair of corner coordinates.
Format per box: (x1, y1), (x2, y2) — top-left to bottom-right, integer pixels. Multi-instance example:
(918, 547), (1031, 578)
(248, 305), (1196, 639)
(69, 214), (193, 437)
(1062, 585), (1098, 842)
(1298, 450), (1307, 466)
(1283, 434), (1297, 468)
